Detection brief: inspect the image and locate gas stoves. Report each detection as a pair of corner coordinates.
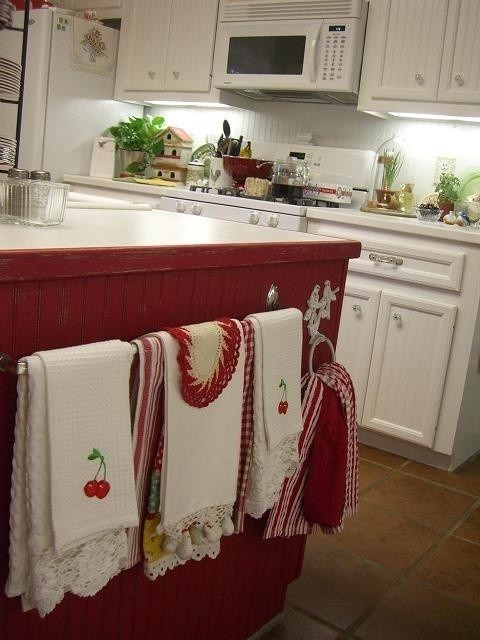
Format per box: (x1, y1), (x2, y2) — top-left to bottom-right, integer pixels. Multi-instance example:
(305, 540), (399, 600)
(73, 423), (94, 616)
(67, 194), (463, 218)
(157, 184), (335, 231)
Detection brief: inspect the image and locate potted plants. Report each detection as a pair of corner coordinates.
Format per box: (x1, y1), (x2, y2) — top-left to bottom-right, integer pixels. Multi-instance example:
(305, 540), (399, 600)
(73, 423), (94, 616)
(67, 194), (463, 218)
(374, 147), (402, 204)
(436, 172), (459, 221)
(109, 116), (167, 176)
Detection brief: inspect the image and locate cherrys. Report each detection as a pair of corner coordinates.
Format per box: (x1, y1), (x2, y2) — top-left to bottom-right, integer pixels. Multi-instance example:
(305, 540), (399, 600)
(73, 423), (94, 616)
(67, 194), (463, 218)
(96, 457), (110, 499)
(284, 384), (288, 414)
(278, 380), (286, 414)
(84, 448), (104, 497)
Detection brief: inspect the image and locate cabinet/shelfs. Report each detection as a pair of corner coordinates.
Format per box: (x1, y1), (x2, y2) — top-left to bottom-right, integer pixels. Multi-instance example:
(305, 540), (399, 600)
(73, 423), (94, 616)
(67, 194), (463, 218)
(357, 0), (479, 124)
(1, 1), (31, 174)
(305, 220), (479, 475)
(112, 1), (218, 110)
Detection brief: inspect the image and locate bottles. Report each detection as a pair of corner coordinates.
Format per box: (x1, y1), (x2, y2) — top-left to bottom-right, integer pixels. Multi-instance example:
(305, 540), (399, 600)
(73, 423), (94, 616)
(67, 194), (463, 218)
(8, 169), (50, 180)
(275, 157), (310, 183)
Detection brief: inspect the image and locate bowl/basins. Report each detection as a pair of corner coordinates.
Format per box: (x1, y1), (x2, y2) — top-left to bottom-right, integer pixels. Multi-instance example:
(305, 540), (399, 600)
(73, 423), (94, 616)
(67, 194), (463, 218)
(416, 208), (444, 223)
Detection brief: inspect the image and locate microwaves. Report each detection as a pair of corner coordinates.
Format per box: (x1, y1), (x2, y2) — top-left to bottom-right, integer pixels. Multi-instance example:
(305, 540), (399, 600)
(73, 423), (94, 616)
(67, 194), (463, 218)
(213, 1), (368, 106)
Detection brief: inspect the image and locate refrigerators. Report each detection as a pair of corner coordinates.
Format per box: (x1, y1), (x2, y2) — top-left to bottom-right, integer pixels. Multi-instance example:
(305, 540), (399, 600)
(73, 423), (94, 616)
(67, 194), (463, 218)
(0, 9), (145, 184)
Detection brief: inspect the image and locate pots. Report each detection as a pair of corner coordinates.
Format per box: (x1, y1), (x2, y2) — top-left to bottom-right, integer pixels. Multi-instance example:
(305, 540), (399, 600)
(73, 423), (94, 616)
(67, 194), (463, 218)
(222, 155), (274, 190)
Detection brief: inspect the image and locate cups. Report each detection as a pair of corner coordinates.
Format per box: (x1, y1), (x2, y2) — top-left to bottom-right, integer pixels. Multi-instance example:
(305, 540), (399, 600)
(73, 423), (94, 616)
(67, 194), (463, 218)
(0, 181), (71, 229)
(186, 162), (204, 186)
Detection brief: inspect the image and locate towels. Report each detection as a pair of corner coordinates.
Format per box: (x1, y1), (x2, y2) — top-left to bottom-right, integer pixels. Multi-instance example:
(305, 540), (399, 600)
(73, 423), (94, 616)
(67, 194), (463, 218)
(131, 320), (255, 581)
(7, 338), (141, 618)
(263, 361), (357, 538)
(243, 307), (303, 519)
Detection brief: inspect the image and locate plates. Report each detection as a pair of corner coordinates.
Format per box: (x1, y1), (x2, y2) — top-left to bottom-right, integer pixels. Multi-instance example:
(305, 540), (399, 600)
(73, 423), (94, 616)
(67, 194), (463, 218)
(0, 135), (17, 172)
(1, 57), (22, 100)
